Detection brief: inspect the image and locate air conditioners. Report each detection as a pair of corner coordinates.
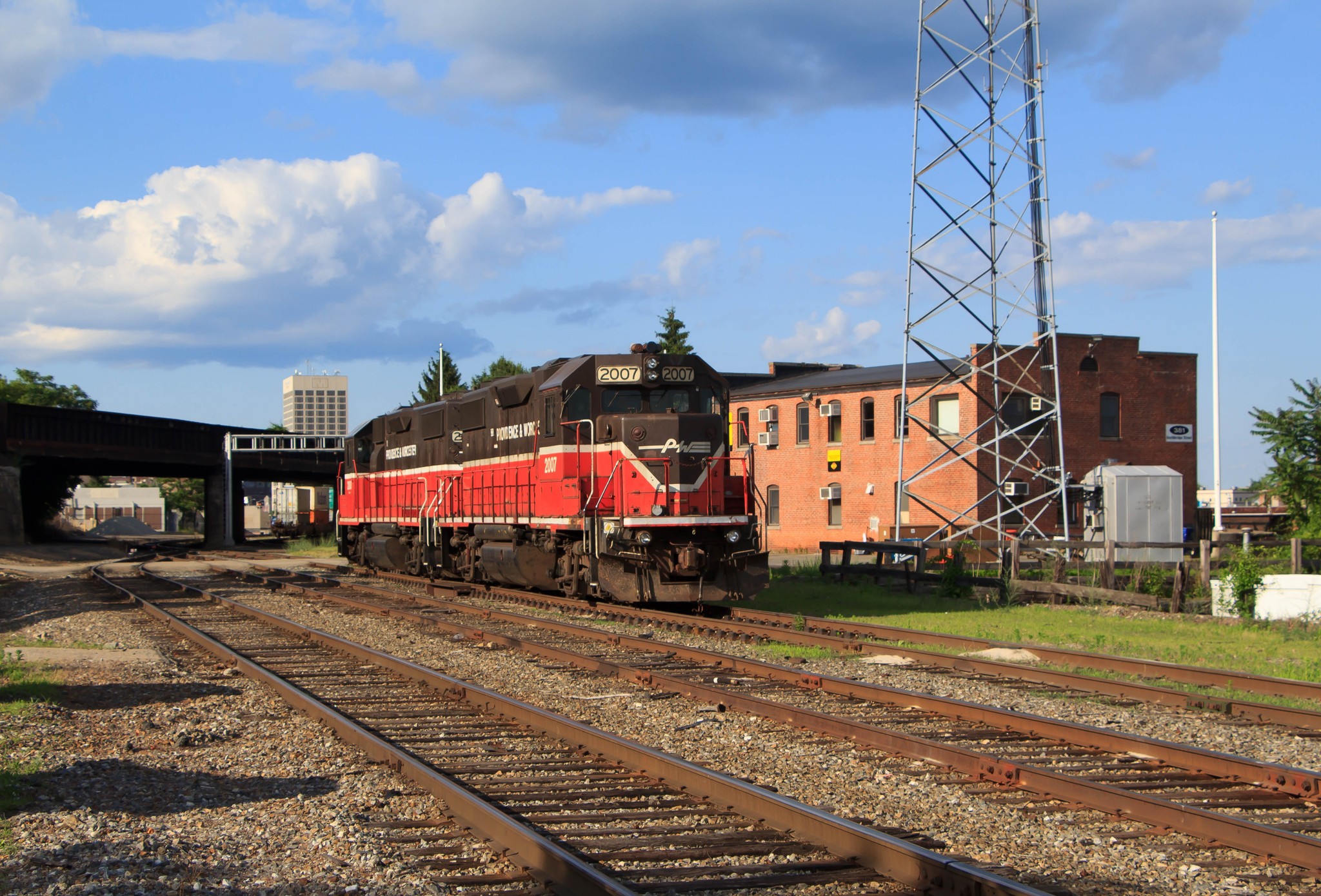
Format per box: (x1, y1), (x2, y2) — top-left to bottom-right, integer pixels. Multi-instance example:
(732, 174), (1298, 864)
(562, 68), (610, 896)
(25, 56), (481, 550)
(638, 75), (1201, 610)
(820, 486), (841, 500)
(759, 408), (778, 422)
(758, 431), (778, 446)
(1003, 481), (1030, 496)
(819, 404), (841, 416)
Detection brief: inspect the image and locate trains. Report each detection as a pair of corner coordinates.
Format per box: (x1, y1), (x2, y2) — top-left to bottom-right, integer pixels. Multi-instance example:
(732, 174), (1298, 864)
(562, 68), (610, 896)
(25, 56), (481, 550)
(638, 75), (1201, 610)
(332, 341), (771, 616)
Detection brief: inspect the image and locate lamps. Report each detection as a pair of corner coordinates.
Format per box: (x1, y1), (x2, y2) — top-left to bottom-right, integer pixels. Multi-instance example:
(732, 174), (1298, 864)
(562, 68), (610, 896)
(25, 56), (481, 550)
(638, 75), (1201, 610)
(1086, 334), (1104, 357)
(801, 392), (817, 402)
